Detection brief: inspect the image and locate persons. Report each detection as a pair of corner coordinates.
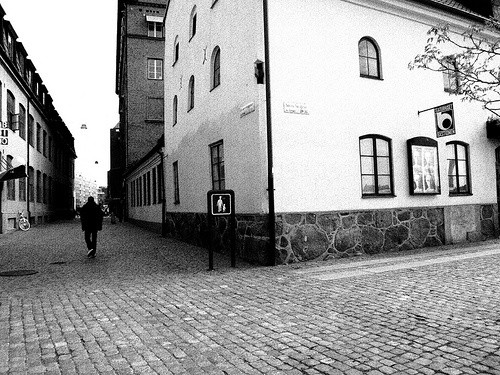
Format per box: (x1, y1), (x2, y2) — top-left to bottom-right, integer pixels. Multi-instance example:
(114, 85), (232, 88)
(79, 197), (104, 258)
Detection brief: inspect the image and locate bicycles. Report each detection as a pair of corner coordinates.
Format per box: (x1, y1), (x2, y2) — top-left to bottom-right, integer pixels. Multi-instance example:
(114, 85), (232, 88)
(17, 210), (31, 231)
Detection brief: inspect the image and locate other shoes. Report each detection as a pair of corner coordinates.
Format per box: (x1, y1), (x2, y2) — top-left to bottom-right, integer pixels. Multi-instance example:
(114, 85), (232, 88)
(87, 249), (93, 257)
(89, 254), (97, 259)
